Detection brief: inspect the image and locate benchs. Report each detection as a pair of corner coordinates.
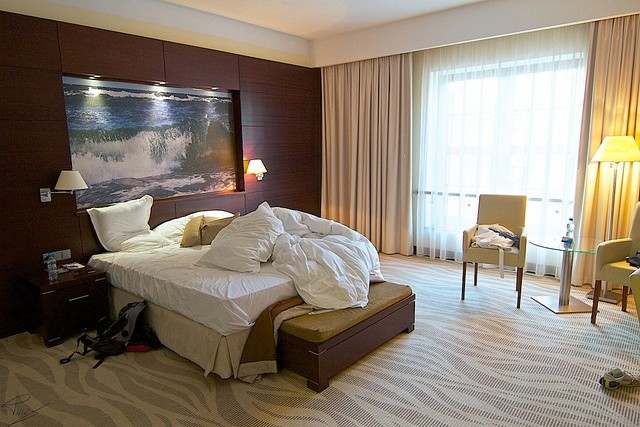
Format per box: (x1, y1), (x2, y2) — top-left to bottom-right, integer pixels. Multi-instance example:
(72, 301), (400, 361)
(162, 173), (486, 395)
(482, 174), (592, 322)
(276, 281), (416, 393)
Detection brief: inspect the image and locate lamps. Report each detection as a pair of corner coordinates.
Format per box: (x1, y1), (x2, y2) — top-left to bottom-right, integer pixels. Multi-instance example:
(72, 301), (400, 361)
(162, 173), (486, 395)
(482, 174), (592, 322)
(585, 136), (640, 304)
(40, 170), (88, 202)
(246, 159), (268, 181)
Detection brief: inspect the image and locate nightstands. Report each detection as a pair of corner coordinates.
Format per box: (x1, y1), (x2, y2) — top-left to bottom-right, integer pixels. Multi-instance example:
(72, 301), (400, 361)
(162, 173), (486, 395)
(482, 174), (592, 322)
(21, 261), (111, 347)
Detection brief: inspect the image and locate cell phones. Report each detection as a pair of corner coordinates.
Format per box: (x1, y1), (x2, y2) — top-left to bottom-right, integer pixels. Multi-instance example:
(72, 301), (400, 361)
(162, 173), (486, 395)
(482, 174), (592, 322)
(86, 266), (95, 274)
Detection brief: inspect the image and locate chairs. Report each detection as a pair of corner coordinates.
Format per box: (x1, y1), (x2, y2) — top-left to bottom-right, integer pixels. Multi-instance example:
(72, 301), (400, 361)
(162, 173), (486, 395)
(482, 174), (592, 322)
(591, 202), (640, 324)
(461, 194), (526, 308)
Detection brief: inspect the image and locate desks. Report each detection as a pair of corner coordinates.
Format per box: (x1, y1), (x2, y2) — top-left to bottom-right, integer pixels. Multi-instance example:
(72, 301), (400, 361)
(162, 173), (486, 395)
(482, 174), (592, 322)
(527, 233), (604, 314)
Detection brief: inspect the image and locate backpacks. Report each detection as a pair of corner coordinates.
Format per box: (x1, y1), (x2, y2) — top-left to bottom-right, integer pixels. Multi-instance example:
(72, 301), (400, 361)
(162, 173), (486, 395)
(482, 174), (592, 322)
(60, 300), (147, 369)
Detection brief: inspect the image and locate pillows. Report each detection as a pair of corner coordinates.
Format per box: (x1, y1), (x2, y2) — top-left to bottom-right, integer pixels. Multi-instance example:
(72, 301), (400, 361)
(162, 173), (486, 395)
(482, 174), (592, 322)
(152, 209), (234, 244)
(86, 195), (171, 253)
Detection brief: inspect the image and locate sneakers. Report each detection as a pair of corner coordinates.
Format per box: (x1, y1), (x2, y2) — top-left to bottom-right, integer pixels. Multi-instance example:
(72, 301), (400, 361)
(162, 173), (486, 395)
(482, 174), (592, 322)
(599, 367), (640, 389)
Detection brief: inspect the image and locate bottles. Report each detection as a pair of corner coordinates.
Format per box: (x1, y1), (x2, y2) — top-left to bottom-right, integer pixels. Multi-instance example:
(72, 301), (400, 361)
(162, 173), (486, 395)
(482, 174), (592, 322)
(566, 217), (575, 239)
(47, 252), (58, 280)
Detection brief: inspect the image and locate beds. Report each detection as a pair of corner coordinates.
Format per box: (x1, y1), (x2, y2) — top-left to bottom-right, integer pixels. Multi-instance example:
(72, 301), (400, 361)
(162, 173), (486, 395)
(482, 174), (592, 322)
(88, 229), (351, 379)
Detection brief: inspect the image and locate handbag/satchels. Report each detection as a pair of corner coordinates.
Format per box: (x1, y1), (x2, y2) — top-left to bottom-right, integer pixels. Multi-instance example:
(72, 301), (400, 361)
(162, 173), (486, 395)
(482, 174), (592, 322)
(470, 223), (519, 250)
